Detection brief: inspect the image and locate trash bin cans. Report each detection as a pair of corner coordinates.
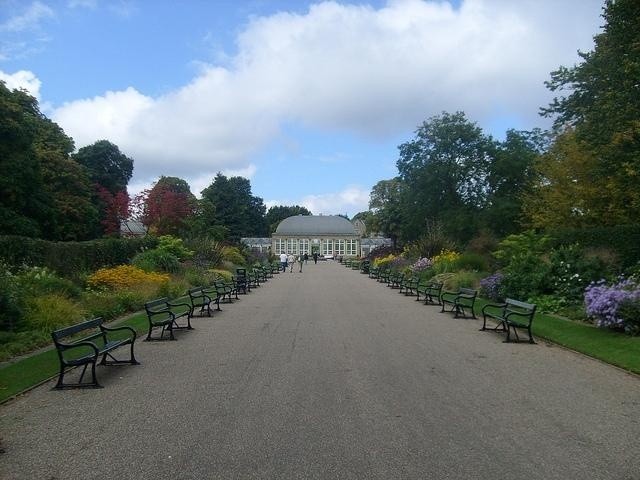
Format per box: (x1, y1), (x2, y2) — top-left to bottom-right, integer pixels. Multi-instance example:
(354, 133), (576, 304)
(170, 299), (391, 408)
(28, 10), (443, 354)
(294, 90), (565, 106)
(236, 269), (246, 287)
(340, 256), (343, 262)
(364, 261), (369, 272)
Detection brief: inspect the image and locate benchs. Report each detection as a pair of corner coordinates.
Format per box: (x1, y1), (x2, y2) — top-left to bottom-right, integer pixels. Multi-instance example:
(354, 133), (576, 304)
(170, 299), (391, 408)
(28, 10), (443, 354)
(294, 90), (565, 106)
(51, 314), (140, 390)
(188, 258), (286, 318)
(478, 295), (538, 347)
(341, 257), (478, 323)
(142, 294), (196, 343)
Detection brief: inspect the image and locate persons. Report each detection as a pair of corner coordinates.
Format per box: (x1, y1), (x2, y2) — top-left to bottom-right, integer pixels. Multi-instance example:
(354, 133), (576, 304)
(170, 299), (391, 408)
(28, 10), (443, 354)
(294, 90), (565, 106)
(288, 253), (296, 272)
(333, 250), (337, 261)
(303, 251), (308, 264)
(296, 251), (304, 273)
(280, 250), (288, 273)
(313, 251), (318, 264)
